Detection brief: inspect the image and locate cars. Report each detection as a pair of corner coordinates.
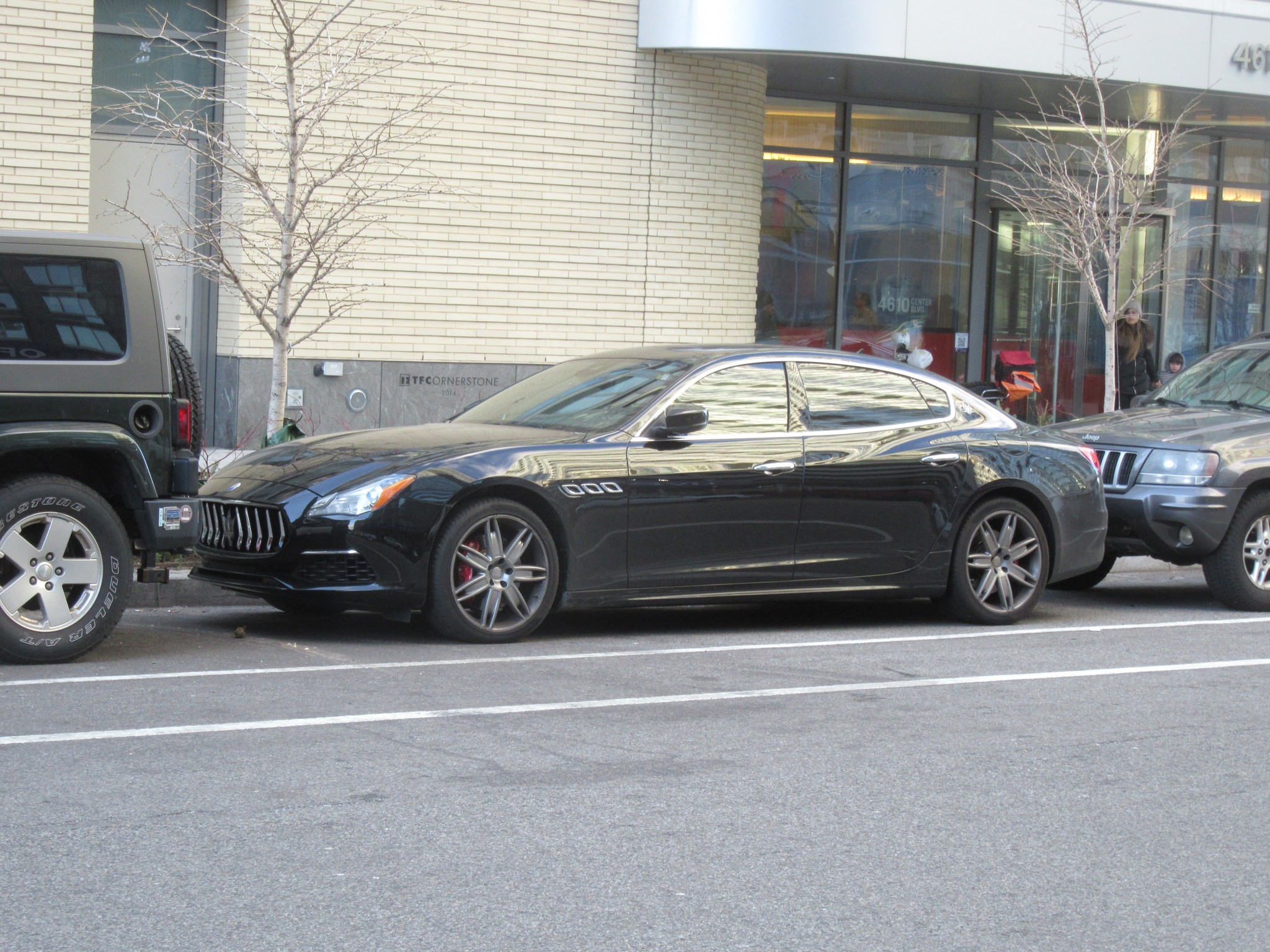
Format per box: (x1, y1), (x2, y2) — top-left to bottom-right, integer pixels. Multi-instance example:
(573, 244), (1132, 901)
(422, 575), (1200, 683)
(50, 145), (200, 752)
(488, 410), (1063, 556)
(194, 344), (1113, 649)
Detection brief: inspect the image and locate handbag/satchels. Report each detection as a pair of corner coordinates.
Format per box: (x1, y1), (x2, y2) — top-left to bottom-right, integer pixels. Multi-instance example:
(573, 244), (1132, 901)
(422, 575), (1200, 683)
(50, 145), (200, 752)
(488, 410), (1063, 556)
(995, 350), (1041, 400)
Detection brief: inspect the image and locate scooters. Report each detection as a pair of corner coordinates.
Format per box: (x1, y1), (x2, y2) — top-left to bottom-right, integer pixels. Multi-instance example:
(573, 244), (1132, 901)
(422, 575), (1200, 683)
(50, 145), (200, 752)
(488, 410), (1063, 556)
(890, 332), (1009, 406)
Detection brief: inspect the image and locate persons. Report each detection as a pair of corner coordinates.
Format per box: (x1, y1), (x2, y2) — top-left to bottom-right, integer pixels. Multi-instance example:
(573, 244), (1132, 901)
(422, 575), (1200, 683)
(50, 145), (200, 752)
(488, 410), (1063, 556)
(1114, 299), (1161, 411)
(1156, 351), (1185, 385)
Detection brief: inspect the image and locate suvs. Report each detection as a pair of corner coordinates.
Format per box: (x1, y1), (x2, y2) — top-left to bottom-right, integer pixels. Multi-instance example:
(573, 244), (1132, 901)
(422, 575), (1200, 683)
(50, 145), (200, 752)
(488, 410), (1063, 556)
(0, 233), (204, 664)
(1039, 333), (1270, 610)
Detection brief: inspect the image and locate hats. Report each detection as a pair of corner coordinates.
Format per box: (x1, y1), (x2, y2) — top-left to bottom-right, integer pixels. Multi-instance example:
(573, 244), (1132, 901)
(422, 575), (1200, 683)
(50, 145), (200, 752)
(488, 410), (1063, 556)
(1123, 300), (1142, 319)
